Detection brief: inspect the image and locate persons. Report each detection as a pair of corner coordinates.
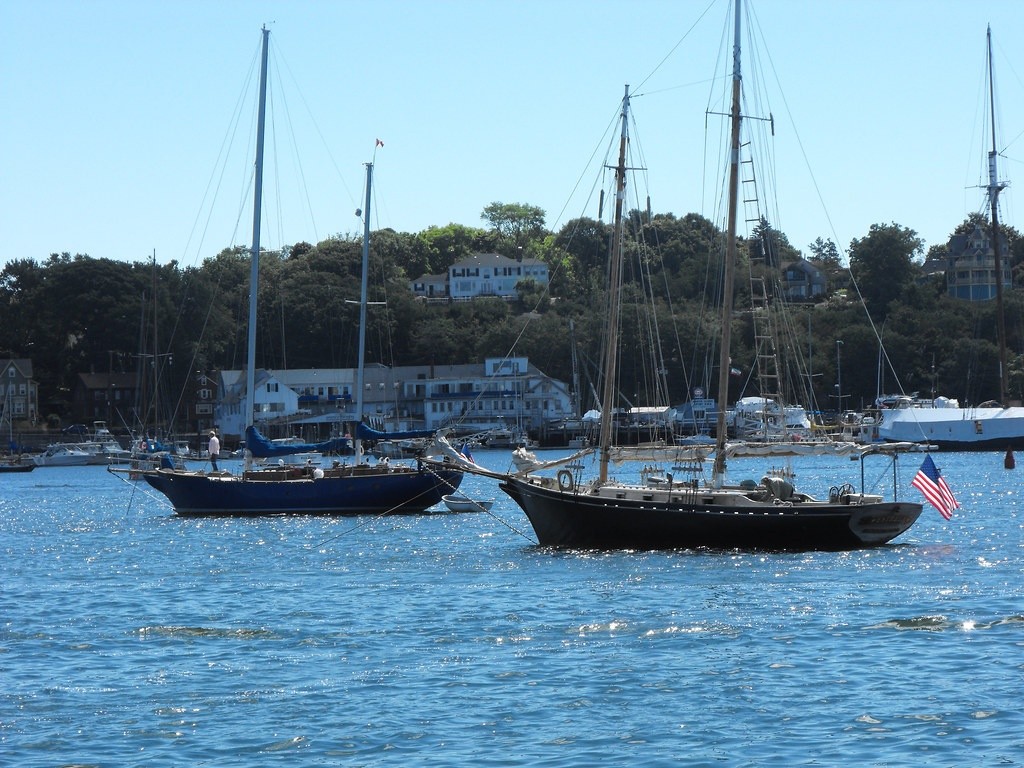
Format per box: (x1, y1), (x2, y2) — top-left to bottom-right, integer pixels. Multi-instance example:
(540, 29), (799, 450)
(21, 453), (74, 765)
(139, 439), (148, 453)
(208, 430), (221, 471)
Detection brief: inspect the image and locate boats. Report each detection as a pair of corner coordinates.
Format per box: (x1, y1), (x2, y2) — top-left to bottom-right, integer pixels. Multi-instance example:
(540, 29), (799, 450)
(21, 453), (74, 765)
(0, 245), (881, 476)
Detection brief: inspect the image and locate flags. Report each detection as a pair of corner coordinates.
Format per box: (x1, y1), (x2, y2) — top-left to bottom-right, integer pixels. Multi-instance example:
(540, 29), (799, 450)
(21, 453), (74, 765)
(461, 442), (475, 470)
(910, 454), (960, 522)
(376, 139), (384, 147)
(730, 367), (742, 377)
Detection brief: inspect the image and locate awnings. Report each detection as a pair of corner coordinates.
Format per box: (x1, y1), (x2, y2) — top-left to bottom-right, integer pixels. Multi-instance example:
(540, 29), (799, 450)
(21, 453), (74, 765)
(298, 395), (318, 409)
(328, 395), (352, 413)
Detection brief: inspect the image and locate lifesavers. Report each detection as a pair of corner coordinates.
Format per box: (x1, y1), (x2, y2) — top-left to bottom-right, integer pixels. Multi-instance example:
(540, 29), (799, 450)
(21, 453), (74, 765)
(557, 470), (574, 491)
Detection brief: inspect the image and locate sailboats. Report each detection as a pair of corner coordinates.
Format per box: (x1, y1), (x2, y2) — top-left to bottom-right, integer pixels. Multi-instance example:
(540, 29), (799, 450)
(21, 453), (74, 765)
(500, 0), (941, 551)
(880, 20), (1024, 450)
(107, 20), (463, 516)
